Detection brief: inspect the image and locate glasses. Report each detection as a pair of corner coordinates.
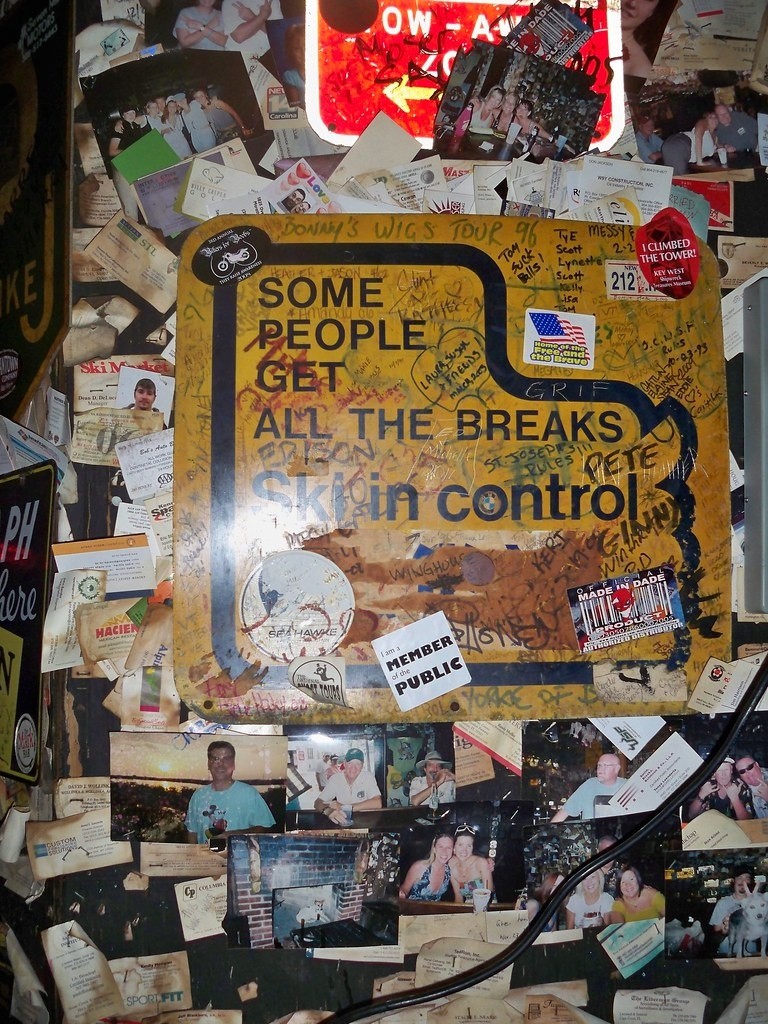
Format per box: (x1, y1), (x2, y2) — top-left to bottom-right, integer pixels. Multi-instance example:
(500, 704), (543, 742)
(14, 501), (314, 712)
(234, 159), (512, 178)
(454, 825), (476, 838)
(209, 755), (233, 765)
(325, 755), (330, 758)
(736, 761), (756, 775)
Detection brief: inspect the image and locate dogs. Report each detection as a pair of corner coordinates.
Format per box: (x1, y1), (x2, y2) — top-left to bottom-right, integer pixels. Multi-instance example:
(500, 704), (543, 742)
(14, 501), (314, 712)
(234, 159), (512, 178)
(730, 892), (768, 962)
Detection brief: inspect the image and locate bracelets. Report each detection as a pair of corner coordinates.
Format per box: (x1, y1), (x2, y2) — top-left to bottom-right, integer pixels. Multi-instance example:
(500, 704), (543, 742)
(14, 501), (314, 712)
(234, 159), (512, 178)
(321, 806), (329, 814)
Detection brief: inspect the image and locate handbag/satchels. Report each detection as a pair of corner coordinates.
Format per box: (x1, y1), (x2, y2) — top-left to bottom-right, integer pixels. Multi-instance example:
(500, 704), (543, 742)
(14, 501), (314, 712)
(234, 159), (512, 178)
(138, 114), (152, 136)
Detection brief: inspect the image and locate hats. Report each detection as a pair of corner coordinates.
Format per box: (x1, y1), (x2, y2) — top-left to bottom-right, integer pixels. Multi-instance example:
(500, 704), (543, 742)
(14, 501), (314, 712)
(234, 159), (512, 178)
(345, 748), (365, 763)
(733, 864), (753, 877)
(329, 754), (339, 762)
(596, 763), (617, 769)
(721, 754), (736, 765)
(174, 93), (186, 102)
(415, 750), (453, 777)
(165, 95), (176, 105)
(118, 102), (140, 114)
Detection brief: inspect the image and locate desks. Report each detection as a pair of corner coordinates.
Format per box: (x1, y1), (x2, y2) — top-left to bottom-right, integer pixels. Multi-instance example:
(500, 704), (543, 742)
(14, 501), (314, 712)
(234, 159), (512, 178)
(437, 129), (542, 164)
(689, 152), (765, 172)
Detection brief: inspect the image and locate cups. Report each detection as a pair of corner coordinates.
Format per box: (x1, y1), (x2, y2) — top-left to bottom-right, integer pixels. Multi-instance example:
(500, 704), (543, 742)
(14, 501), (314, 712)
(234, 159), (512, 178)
(473, 889), (492, 913)
(340, 805), (352, 827)
(583, 915), (600, 928)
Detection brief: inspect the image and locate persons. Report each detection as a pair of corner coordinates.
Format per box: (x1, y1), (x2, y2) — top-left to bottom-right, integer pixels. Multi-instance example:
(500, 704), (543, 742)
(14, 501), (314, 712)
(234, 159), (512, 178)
(105, 1), (768, 958)
(184, 741), (276, 844)
